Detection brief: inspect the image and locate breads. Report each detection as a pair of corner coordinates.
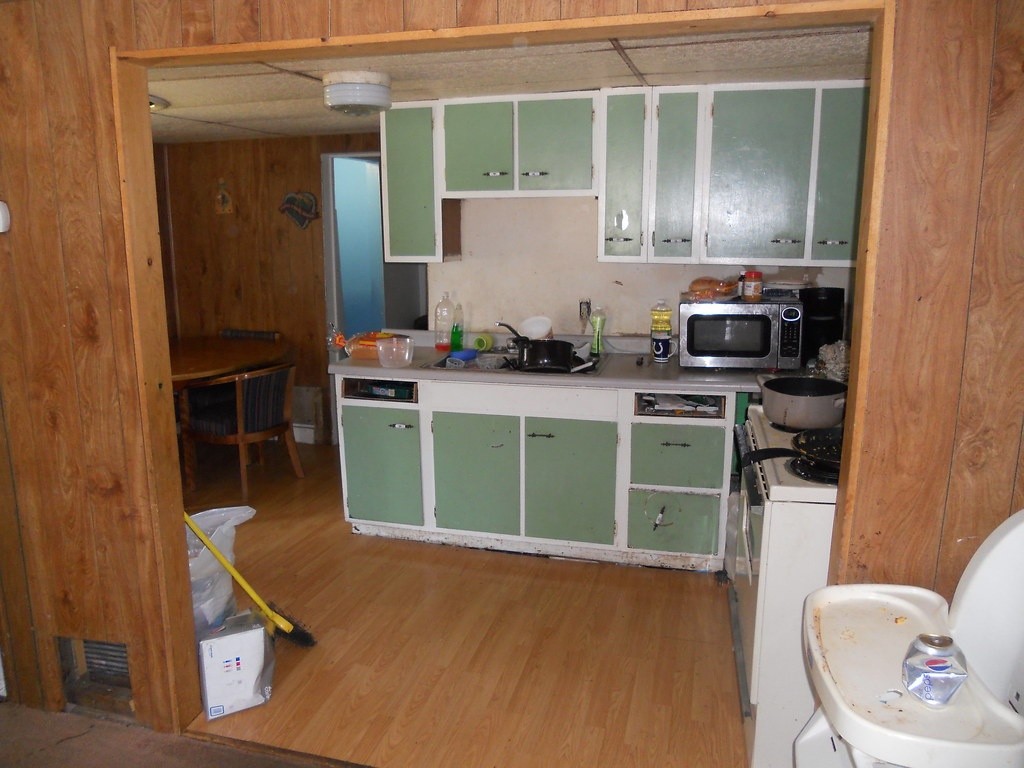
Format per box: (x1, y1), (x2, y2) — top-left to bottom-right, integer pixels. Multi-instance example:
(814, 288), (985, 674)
(690, 276), (737, 293)
(346, 331), (411, 360)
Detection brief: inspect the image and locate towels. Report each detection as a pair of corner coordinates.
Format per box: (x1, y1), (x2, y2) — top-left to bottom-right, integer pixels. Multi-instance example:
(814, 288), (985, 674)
(723, 491), (740, 584)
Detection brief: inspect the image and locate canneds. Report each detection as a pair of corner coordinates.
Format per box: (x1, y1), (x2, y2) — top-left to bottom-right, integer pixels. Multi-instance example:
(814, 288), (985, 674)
(738, 271), (763, 302)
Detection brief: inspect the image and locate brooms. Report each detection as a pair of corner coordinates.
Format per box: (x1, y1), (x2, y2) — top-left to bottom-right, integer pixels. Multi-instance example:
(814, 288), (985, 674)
(184, 509), (318, 648)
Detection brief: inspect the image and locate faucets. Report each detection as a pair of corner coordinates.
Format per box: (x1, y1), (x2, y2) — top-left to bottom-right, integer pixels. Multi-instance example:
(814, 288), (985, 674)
(493, 321), (523, 354)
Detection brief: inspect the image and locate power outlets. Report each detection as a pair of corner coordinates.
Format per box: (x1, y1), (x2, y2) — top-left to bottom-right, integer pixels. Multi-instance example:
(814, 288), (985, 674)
(579, 299), (591, 318)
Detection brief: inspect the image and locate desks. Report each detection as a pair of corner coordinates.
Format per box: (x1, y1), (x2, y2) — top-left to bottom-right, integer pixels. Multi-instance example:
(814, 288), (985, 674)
(170, 337), (294, 493)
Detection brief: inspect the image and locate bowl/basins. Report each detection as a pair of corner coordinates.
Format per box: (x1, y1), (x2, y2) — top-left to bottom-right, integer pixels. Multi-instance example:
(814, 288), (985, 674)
(376, 338), (415, 368)
(449, 349), (480, 360)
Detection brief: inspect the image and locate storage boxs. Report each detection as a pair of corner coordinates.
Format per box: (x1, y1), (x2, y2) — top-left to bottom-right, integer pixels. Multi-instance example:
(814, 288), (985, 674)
(199, 606), (274, 721)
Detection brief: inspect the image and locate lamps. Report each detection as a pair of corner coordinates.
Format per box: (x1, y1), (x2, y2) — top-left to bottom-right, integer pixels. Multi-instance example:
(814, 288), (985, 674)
(323, 70), (392, 117)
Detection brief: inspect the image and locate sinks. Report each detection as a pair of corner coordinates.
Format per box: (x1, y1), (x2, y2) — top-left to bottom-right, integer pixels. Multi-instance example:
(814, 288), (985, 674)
(431, 348), (609, 379)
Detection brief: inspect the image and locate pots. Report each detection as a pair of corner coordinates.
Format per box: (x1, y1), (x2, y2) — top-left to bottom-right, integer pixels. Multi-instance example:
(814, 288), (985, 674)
(496, 321), (574, 373)
(755, 374), (848, 430)
(737, 429), (843, 471)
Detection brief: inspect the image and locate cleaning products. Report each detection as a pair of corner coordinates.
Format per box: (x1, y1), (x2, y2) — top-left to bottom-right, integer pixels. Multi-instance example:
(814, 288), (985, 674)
(450, 301), (463, 352)
(589, 307), (606, 357)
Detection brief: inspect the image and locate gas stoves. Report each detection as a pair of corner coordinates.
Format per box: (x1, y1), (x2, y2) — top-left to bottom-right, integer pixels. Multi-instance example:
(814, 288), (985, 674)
(745, 404), (844, 503)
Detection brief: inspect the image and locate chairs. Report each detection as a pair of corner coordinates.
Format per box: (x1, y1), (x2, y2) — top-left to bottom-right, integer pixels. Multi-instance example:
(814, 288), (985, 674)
(175, 329), (304, 497)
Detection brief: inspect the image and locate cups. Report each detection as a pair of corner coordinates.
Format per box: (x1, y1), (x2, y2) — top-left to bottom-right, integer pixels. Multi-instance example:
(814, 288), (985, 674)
(517, 316), (553, 340)
(652, 336), (677, 363)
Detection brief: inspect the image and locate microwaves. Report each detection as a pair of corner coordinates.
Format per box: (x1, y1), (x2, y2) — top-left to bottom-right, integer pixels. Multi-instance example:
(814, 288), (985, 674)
(679, 289), (803, 374)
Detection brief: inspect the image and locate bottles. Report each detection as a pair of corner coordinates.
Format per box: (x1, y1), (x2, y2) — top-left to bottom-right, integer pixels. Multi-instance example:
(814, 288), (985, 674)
(650, 299), (672, 360)
(590, 306), (607, 357)
(737, 271), (747, 299)
(472, 335), (494, 351)
(450, 303), (463, 352)
(434, 292), (454, 352)
(744, 272), (763, 302)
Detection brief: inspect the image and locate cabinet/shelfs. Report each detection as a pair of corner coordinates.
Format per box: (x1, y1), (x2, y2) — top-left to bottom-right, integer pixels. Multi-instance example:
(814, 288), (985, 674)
(379, 78), (868, 268)
(334, 375), (735, 569)
